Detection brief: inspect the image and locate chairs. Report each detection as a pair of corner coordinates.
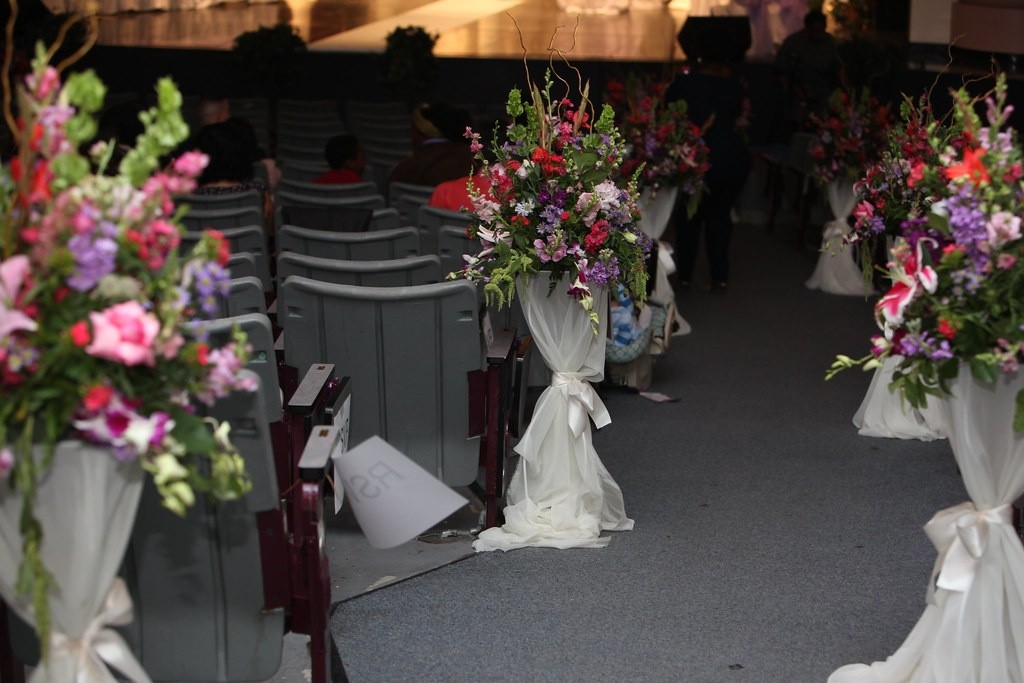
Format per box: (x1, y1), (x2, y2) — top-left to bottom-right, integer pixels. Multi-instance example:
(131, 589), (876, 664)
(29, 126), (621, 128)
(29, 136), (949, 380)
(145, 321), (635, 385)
(102, 97), (552, 683)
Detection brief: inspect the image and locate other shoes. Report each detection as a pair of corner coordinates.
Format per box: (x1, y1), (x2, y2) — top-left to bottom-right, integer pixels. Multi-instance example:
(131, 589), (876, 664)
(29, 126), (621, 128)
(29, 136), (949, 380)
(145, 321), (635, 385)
(674, 281), (689, 295)
(711, 281), (728, 295)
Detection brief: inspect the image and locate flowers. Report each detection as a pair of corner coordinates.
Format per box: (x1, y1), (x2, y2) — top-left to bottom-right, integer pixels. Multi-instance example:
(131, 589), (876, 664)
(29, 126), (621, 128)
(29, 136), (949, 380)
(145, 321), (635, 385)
(793, 28), (1024, 435)
(446, 12), (717, 341)
(0, 42), (264, 667)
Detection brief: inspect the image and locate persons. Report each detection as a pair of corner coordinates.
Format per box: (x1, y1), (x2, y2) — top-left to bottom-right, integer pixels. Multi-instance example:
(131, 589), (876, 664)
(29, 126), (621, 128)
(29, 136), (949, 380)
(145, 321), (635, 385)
(659, 47), (754, 296)
(159, 83), (523, 218)
(762, 8), (871, 206)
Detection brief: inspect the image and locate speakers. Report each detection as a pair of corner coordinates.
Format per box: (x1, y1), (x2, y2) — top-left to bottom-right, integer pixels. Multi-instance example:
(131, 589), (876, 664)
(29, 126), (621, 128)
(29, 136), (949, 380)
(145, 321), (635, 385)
(677, 16), (752, 59)
